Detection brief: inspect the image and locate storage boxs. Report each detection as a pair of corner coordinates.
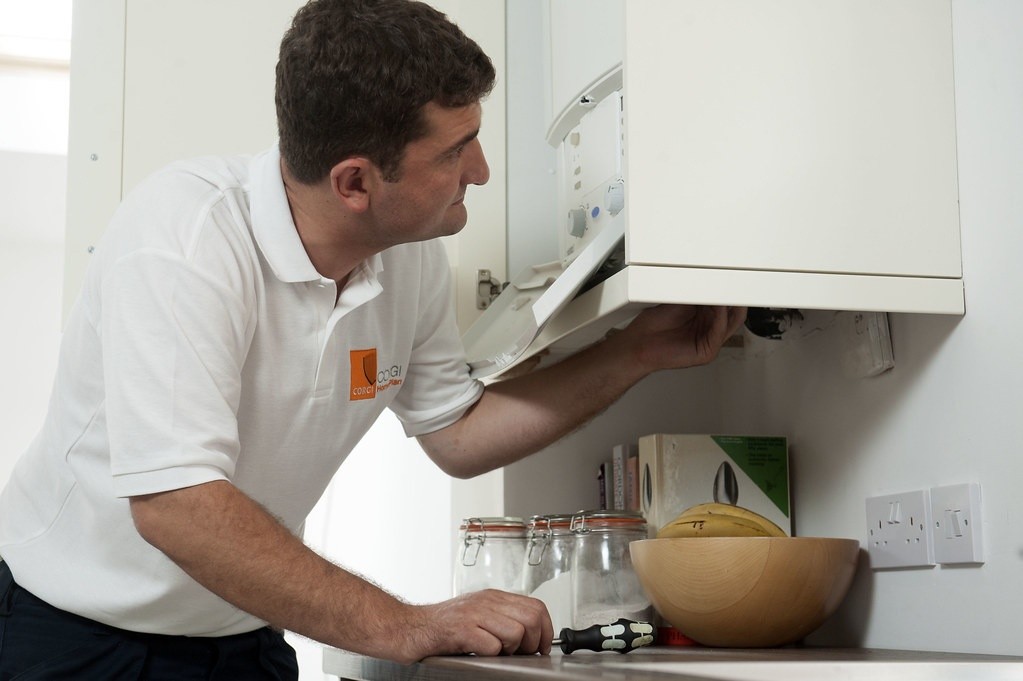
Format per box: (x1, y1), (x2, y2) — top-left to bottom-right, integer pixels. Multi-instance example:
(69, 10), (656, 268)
(637, 433), (791, 538)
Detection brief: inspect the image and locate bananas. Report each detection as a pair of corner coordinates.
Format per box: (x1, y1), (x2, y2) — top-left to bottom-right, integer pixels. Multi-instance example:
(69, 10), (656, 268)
(656, 503), (787, 538)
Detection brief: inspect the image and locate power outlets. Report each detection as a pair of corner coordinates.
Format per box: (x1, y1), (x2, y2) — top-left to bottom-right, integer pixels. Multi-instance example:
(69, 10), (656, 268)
(864, 490), (933, 572)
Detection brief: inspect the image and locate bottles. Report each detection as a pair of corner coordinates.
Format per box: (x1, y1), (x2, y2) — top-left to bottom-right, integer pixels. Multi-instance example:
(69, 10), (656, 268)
(454, 518), (532, 599)
(570, 509), (656, 645)
(522, 514), (575, 644)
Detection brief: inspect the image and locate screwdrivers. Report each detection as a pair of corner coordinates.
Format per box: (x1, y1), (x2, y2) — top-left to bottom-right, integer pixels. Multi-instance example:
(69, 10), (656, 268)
(470, 616), (657, 657)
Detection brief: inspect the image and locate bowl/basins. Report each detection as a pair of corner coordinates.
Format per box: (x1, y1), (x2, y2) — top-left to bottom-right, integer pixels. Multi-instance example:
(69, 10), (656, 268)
(628, 538), (859, 647)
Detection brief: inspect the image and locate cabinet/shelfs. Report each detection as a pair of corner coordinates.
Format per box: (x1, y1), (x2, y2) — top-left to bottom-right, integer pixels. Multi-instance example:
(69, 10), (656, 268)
(68, 0), (964, 381)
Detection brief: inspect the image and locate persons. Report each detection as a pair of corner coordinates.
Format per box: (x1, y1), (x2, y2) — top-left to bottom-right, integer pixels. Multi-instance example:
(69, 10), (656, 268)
(0, 0), (748, 681)
